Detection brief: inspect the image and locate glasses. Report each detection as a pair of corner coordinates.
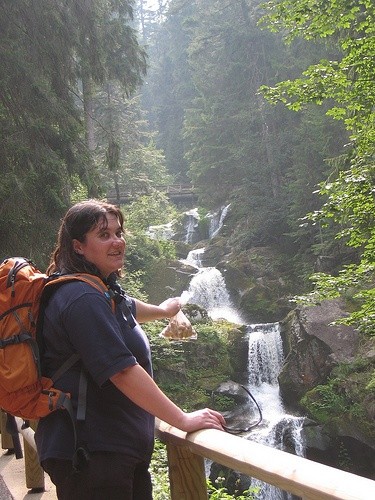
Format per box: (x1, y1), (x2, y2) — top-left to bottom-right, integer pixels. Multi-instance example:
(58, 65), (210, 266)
(211, 385), (263, 435)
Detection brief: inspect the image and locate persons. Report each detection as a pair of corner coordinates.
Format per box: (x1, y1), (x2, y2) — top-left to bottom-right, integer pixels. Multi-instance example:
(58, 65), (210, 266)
(32, 200), (226, 499)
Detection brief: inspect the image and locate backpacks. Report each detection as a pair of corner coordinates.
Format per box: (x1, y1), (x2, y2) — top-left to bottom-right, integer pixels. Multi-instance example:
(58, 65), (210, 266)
(0, 256), (117, 420)
